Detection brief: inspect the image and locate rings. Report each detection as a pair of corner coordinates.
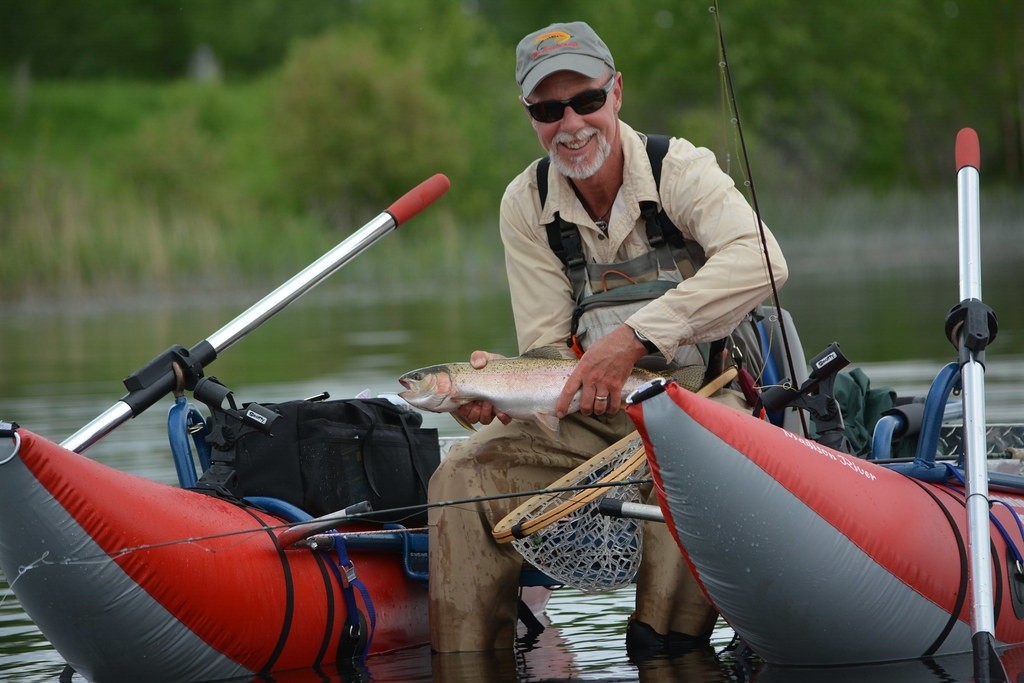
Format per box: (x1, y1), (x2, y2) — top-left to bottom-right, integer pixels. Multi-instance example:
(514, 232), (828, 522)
(596, 396), (607, 401)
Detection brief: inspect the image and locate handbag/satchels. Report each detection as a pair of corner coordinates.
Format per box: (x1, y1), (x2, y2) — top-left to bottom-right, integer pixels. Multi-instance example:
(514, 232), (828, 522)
(192, 375), (441, 527)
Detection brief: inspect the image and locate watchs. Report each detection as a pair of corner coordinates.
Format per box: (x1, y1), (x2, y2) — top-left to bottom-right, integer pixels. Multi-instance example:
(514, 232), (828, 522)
(633, 328), (660, 355)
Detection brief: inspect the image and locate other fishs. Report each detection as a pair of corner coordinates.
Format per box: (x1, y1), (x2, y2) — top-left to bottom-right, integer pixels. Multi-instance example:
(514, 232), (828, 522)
(396, 356), (665, 434)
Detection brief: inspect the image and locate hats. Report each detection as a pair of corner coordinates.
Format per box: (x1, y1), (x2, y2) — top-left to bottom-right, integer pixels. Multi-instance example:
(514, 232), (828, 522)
(516, 21), (615, 98)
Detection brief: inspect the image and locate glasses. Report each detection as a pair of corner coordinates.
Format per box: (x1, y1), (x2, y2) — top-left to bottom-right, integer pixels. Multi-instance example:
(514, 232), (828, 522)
(522, 74), (615, 123)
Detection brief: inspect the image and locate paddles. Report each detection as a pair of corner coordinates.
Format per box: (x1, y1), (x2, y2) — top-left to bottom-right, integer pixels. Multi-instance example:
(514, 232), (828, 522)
(57, 172), (450, 457)
(954, 126), (1010, 683)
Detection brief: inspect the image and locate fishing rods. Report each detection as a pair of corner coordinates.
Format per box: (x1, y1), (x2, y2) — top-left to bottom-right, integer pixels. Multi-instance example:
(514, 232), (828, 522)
(714, 0), (852, 451)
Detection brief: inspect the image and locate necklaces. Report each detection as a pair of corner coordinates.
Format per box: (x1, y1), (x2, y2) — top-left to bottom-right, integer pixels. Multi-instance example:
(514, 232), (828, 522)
(594, 204), (612, 231)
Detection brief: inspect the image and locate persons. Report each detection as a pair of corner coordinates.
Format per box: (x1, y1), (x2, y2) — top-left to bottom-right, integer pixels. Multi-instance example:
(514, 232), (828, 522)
(429, 22), (788, 653)
